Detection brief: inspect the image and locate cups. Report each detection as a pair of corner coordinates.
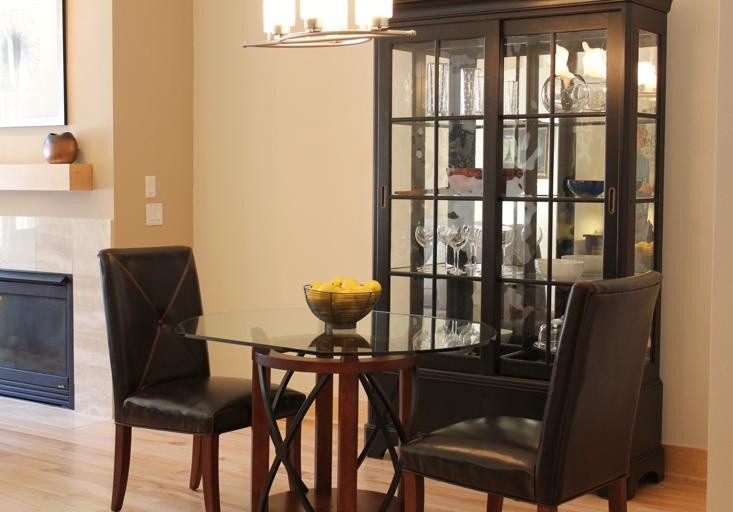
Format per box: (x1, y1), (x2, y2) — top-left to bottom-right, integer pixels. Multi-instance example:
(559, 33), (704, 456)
(423, 60), (519, 119)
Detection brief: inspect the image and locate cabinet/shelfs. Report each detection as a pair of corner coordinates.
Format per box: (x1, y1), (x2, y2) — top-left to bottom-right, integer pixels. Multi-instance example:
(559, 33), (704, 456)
(364, 1), (671, 502)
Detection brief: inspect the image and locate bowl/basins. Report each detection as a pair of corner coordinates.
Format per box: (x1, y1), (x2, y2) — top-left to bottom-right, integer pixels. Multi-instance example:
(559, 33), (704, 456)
(534, 254), (582, 283)
(560, 252), (603, 274)
(566, 178), (607, 199)
(499, 329), (513, 344)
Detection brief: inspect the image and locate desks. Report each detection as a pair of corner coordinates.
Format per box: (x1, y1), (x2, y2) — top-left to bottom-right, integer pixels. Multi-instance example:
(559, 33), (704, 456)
(172, 306), (500, 512)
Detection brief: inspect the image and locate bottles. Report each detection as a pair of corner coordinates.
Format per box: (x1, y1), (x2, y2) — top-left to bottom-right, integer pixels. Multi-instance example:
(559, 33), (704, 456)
(541, 75), (588, 114)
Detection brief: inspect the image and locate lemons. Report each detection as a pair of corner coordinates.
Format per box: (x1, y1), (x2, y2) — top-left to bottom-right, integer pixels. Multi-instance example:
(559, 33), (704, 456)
(306, 275), (382, 322)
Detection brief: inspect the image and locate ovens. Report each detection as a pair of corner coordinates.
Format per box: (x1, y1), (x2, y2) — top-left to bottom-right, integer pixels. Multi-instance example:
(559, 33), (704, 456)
(0, 268), (71, 411)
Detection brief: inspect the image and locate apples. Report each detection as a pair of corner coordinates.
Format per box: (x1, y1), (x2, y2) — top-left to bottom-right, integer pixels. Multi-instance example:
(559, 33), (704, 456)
(42, 132), (78, 163)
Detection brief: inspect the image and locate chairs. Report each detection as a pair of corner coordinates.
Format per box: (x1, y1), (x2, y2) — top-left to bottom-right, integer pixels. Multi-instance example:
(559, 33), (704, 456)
(398, 269), (663, 510)
(96, 247), (306, 512)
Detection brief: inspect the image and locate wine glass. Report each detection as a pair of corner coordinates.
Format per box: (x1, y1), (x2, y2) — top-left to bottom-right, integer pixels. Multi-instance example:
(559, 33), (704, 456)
(409, 222), (516, 280)
(413, 319), (482, 354)
